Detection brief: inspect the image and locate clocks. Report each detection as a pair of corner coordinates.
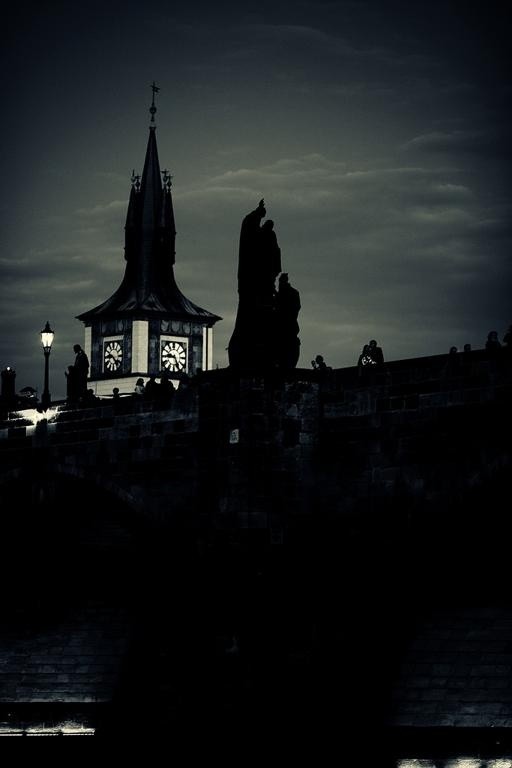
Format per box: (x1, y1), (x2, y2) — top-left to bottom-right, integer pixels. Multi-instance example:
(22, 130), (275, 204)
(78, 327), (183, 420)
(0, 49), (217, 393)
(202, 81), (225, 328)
(103, 340), (125, 374)
(161, 341), (188, 373)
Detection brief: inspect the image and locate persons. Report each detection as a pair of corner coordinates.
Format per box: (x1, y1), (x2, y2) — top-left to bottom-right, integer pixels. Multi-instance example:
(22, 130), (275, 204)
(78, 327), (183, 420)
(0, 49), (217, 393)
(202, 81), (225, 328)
(464, 344), (471, 352)
(64, 344), (89, 400)
(311, 355), (332, 370)
(113, 368), (204, 398)
(450, 346), (457, 353)
(358, 340), (384, 366)
(486, 331), (502, 349)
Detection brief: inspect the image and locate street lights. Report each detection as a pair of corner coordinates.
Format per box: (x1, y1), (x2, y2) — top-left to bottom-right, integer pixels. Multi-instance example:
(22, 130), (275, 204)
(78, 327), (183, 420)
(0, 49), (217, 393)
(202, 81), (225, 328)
(39, 321), (55, 403)
